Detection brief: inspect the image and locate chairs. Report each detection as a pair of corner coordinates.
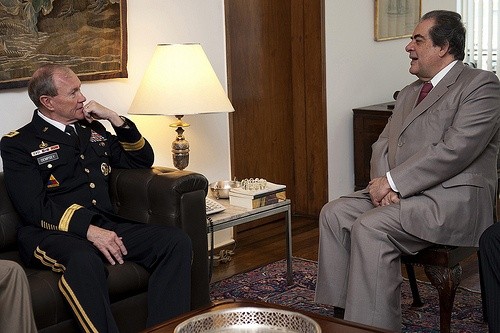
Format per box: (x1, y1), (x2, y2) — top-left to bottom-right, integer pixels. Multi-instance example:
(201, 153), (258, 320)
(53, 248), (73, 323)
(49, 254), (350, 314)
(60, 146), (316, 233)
(400, 185), (500, 333)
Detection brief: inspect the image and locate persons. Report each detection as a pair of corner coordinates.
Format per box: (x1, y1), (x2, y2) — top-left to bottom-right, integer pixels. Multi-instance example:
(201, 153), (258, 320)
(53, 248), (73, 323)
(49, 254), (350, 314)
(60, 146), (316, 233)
(0, 65), (193, 333)
(314, 10), (500, 332)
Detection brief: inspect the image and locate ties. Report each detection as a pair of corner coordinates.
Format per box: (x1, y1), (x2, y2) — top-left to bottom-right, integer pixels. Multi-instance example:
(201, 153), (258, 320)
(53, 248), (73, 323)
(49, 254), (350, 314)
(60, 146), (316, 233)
(417, 82), (433, 105)
(65, 125), (77, 137)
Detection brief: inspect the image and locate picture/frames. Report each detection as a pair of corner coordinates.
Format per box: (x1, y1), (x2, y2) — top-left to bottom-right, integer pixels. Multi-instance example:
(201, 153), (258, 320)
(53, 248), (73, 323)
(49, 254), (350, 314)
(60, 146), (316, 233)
(0, 0), (128, 91)
(374, 0), (423, 43)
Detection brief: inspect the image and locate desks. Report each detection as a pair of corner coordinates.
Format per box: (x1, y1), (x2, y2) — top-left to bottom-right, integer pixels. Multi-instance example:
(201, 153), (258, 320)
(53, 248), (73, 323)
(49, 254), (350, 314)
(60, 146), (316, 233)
(205, 185), (293, 286)
(139, 299), (399, 333)
(352, 101), (398, 192)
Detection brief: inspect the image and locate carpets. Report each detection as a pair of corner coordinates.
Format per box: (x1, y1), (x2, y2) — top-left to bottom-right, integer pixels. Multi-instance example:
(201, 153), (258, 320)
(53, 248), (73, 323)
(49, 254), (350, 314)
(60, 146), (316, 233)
(208, 254), (489, 333)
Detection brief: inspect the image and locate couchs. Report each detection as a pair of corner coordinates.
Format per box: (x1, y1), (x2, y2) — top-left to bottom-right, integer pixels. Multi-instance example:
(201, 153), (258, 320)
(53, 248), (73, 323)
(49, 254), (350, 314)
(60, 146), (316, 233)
(0, 166), (208, 333)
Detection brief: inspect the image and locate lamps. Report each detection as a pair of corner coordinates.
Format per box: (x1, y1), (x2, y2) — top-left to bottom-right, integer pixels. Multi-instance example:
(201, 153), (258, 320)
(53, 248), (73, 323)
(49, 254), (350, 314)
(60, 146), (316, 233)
(127, 43), (236, 170)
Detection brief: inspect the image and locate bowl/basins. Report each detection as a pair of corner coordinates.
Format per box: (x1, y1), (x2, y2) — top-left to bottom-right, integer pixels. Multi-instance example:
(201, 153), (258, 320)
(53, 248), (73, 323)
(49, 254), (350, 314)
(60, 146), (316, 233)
(209, 177), (241, 200)
